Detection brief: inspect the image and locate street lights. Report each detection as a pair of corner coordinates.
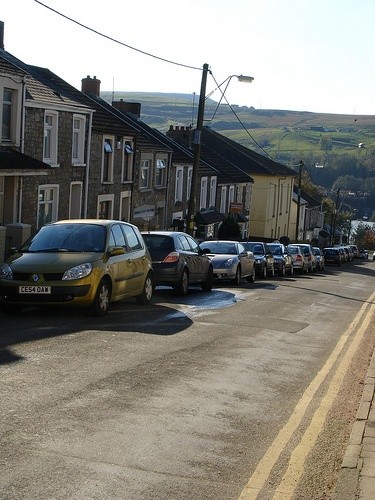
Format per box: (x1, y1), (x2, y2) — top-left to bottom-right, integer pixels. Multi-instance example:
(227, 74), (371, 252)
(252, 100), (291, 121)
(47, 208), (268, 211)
(185, 63), (255, 237)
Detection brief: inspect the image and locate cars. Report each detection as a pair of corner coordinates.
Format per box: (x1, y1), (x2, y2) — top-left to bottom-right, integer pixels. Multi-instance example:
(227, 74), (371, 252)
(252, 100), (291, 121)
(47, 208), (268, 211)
(312, 246), (326, 272)
(289, 243), (318, 273)
(125, 230), (214, 297)
(239, 241), (275, 279)
(324, 242), (369, 267)
(284, 245), (309, 274)
(267, 242), (294, 277)
(199, 240), (257, 285)
(0, 219), (156, 317)
